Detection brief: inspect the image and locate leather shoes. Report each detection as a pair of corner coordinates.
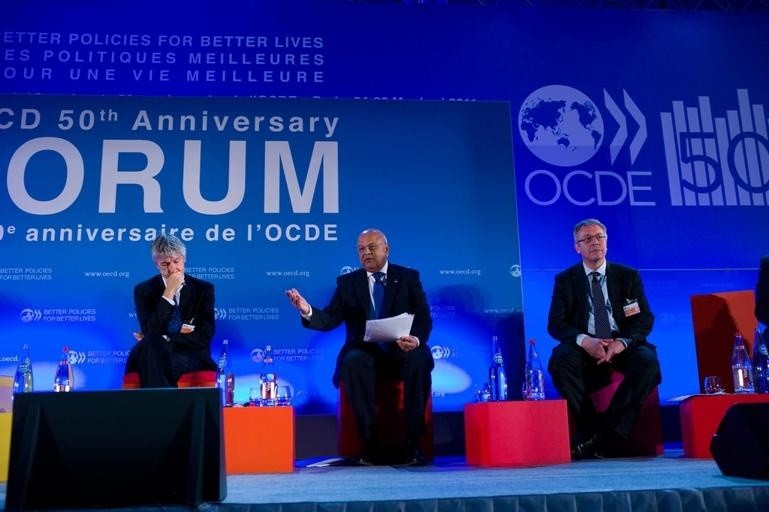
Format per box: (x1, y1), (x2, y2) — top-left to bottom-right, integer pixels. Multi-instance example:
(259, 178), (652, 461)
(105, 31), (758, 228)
(405, 453), (427, 467)
(572, 432), (600, 460)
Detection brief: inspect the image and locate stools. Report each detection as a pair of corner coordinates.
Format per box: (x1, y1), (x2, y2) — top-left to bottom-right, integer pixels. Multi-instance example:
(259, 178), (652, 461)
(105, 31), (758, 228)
(334, 371), (435, 463)
(122, 366), (220, 390)
(558, 350), (665, 460)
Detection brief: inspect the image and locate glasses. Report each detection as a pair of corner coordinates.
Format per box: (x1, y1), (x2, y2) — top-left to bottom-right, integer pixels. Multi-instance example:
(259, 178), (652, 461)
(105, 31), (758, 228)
(576, 233), (606, 242)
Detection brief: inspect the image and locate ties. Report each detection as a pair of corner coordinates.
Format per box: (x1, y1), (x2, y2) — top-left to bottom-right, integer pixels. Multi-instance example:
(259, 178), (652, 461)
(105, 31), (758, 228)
(169, 296), (182, 335)
(373, 271), (387, 351)
(592, 272), (611, 339)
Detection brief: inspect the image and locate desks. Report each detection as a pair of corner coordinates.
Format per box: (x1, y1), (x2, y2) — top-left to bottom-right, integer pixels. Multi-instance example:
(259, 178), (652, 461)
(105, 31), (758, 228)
(461, 397), (571, 467)
(219, 407), (295, 478)
(680, 389), (765, 460)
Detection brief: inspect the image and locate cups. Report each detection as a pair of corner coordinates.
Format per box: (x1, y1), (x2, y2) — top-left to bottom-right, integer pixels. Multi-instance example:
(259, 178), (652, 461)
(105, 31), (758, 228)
(277, 384), (292, 407)
(474, 383), (490, 402)
(249, 387), (260, 407)
(522, 381), (528, 401)
(703, 374), (722, 395)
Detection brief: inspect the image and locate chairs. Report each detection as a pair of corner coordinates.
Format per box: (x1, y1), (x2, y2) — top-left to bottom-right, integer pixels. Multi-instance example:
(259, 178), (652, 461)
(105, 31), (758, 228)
(687, 288), (763, 396)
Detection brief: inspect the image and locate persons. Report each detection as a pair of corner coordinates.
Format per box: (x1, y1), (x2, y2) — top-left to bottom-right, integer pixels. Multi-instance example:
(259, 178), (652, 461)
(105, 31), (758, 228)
(288, 229), (434, 467)
(547, 218), (661, 461)
(755, 257), (769, 351)
(125, 235), (222, 388)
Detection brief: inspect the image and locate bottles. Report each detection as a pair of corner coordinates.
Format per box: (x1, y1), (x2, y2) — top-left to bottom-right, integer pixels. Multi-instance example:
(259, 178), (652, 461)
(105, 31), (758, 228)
(259, 343), (277, 407)
(11, 343), (34, 395)
(525, 338), (545, 401)
(489, 335), (509, 401)
(53, 346), (74, 393)
(750, 326), (769, 395)
(217, 339), (234, 407)
(731, 331), (757, 395)
